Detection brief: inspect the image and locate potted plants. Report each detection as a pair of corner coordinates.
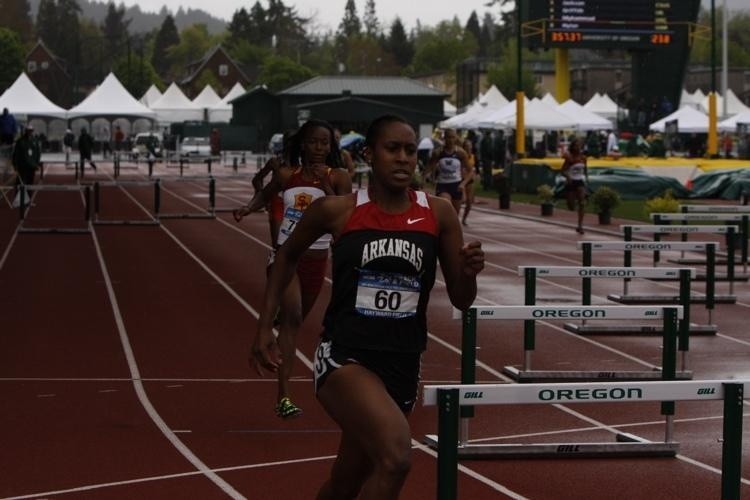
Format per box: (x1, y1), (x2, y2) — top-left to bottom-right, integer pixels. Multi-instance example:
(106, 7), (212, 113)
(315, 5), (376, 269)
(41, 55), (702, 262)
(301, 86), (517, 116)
(492, 172), (514, 209)
(641, 187), (682, 236)
(535, 183), (557, 217)
(591, 184), (624, 226)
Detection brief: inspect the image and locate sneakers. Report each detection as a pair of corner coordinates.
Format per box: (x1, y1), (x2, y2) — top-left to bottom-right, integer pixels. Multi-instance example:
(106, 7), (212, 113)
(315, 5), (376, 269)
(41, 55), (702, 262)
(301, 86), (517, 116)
(273, 397), (303, 418)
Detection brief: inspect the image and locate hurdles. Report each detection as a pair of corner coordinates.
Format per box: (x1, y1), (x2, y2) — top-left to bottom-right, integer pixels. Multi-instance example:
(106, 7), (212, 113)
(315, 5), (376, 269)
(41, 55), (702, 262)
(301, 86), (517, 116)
(207, 176), (266, 212)
(94, 181), (161, 225)
(504, 265), (697, 383)
(608, 224), (740, 304)
(564, 240), (720, 335)
(156, 178), (216, 219)
(40, 154), (277, 187)
(666, 204), (750, 265)
(17, 184), (94, 234)
(422, 379), (750, 500)
(646, 212), (750, 281)
(424, 304), (684, 460)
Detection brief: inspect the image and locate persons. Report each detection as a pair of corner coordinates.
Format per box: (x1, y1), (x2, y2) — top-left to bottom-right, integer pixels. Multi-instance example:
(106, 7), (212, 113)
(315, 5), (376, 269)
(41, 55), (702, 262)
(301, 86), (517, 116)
(210, 128), (221, 163)
(115, 127), (124, 150)
(103, 127), (112, 159)
(163, 126), (169, 140)
(12, 125), (38, 208)
(64, 129), (75, 166)
(78, 128), (96, 177)
(0, 108), (17, 157)
